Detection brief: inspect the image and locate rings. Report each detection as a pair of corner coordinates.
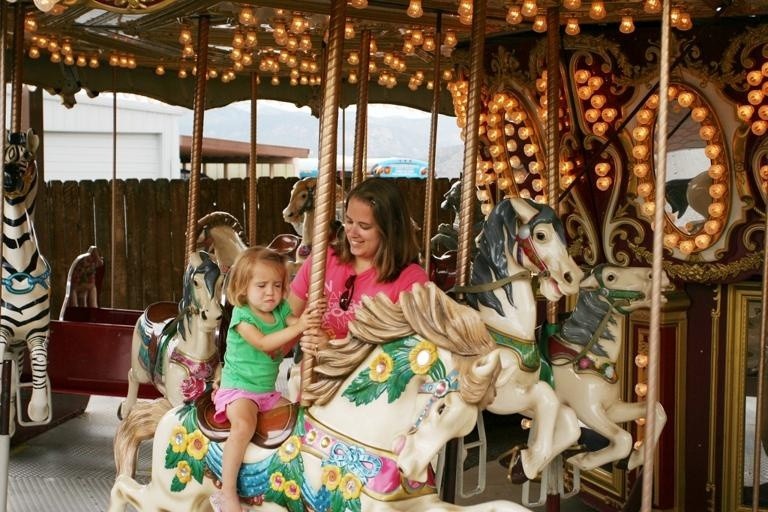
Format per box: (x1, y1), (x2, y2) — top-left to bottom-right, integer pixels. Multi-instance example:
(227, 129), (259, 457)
(315, 343), (318, 351)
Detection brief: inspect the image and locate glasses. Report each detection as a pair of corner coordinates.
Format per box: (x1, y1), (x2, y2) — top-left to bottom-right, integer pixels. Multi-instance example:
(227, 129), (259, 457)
(340, 274), (356, 312)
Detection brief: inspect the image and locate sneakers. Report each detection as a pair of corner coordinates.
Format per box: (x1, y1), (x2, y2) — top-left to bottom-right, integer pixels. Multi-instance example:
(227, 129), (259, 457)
(209, 490), (247, 512)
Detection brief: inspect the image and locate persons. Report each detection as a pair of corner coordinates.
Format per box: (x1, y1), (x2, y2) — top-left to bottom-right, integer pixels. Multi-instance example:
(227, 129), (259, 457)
(211, 245), (331, 511)
(284, 172), (427, 403)
